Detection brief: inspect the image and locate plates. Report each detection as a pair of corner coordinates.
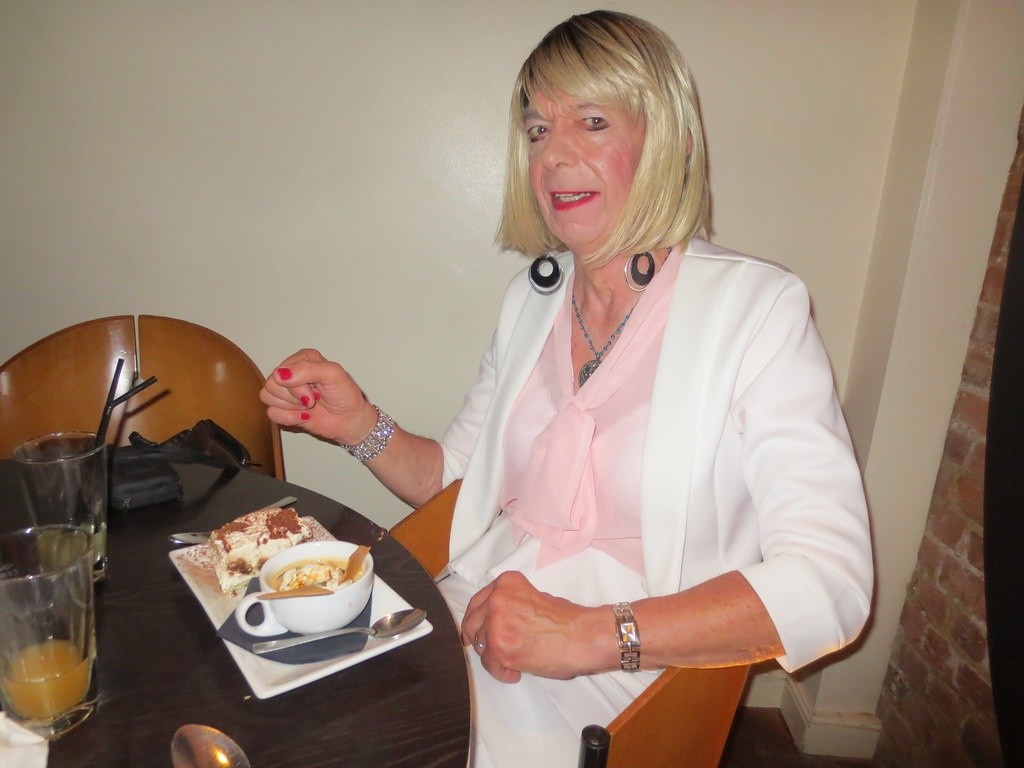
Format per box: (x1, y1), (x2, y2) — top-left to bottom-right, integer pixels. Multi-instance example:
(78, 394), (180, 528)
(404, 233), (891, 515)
(169, 515), (433, 699)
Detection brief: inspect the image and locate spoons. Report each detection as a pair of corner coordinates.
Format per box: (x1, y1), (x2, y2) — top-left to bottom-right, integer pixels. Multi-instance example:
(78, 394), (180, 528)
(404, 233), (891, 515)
(171, 723), (251, 768)
(252, 608), (427, 654)
(168, 495), (298, 545)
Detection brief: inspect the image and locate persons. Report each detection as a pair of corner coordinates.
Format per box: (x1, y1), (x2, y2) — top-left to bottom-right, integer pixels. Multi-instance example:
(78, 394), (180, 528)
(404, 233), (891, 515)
(261, 9), (872, 768)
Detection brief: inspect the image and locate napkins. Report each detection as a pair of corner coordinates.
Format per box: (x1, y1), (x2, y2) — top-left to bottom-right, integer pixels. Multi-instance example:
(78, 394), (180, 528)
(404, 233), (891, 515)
(0, 711), (50, 768)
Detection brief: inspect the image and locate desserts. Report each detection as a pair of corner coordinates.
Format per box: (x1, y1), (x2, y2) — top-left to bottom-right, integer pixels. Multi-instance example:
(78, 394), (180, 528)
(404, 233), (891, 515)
(208, 506), (312, 590)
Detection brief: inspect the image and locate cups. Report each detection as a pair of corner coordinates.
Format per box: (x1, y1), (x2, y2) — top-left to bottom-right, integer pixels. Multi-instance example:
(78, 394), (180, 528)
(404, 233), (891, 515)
(234, 539), (374, 637)
(0, 525), (99, 743)
(11, 430), (107, 581)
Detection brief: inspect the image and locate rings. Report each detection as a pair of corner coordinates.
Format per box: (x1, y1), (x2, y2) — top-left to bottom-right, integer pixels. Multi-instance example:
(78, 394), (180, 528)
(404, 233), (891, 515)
(474, 634), (486, 648)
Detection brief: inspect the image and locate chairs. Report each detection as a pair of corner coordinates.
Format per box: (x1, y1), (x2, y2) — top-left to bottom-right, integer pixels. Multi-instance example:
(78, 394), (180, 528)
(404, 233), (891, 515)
(389, 479), (753, 768)
(0, 313), (287, 480)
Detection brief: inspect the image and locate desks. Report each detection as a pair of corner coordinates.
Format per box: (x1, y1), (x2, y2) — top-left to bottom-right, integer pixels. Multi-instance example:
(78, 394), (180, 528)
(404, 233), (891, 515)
(0, 459), (473, 768)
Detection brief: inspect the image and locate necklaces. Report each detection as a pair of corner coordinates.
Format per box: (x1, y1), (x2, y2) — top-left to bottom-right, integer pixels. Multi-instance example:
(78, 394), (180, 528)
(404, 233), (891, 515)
(570, 250), (670, 389)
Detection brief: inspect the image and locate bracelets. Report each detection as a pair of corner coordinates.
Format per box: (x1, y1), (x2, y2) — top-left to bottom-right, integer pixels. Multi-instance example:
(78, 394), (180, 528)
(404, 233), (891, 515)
(340, 405), (394, 460)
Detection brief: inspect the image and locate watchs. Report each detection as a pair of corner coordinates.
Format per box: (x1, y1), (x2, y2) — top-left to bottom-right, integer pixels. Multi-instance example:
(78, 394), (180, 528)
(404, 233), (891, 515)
(613, 601), (641, 673)
